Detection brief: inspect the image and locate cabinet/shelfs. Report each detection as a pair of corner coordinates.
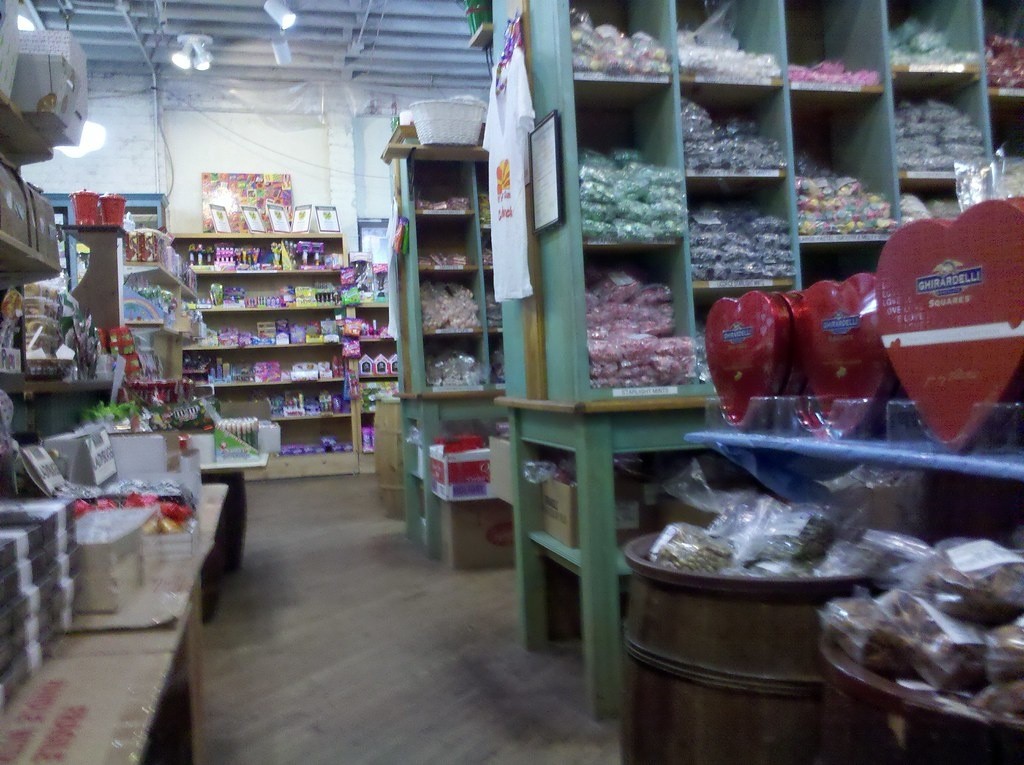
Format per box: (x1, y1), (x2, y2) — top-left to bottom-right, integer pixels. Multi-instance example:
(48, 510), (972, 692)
(355, 302), (398, 475)
(382, 124), (506, 561)
(170, 233), (359, 481)
(491, 0), (1024, 721)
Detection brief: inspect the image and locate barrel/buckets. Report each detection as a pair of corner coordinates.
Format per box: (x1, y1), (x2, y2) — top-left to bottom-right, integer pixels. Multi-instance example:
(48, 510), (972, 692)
(617, 530), (876, 765)
(819, 590), (1024, 765)
(69, 190), (99, 226)
(99, 194), (127, 228)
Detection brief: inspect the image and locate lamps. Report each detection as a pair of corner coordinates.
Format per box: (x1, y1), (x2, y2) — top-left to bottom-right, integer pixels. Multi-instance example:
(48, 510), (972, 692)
(271, 36), (292, 67)
(172, 34), (214, 70)
(264, 0), (297, 30)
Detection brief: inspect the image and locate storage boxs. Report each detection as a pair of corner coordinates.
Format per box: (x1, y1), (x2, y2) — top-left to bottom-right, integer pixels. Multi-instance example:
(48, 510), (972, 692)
(12, 53), (81, 131)
(543, 475), (642, 550)
(428, 441), (498, 502)
